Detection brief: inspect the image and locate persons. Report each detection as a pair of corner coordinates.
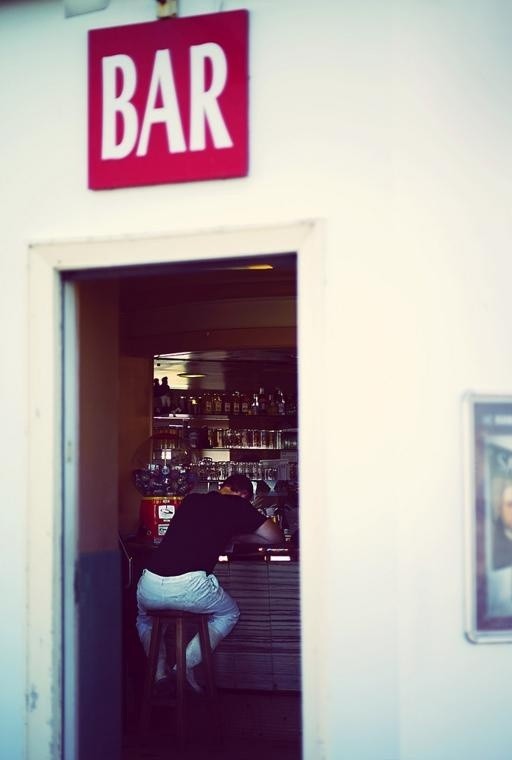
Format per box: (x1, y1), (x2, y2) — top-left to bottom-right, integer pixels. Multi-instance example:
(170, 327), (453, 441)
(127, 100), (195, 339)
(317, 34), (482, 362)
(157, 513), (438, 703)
(491, 479), (512, 572)
(136, 473), (286, 692)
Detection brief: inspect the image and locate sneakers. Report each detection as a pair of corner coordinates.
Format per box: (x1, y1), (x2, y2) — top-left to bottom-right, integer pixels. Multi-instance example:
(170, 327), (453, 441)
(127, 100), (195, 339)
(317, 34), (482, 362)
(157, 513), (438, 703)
(153, 663), (204, 696)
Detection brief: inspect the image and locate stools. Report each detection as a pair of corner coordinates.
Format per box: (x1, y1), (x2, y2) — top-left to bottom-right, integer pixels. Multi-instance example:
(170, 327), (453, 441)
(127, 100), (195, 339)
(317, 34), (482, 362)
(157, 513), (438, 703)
(128, 608), (222, 751)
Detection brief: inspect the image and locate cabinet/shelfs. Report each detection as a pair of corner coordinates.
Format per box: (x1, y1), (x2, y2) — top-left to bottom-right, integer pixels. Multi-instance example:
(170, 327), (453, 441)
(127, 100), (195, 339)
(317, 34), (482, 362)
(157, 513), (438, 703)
(150, 410), (297, 486)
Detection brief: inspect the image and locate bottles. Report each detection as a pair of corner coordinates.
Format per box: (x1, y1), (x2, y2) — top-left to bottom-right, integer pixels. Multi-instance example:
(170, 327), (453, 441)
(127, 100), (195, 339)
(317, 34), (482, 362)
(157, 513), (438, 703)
(173, 387), (298, 416)
(153, 426), (183, 448)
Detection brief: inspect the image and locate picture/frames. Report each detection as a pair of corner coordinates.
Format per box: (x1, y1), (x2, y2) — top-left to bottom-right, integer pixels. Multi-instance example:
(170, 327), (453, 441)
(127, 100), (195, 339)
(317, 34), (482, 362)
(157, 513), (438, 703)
(462, 392), (512, 646)
